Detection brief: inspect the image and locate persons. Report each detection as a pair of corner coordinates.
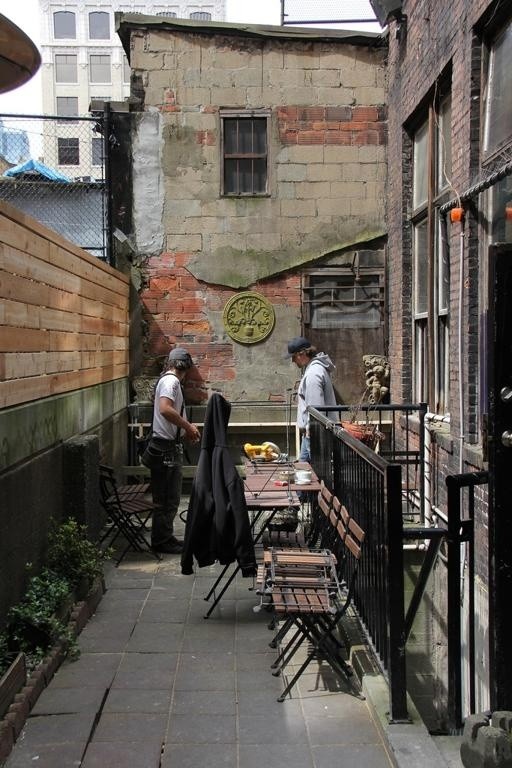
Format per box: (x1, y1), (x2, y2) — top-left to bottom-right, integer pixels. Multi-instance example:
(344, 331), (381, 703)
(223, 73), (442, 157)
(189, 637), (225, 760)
(149, 347), (202, 555)
(284, 336), (340, 503)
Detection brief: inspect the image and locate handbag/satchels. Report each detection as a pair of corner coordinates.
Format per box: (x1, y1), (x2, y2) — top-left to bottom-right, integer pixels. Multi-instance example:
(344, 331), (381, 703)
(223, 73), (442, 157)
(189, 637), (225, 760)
(140, 441), (174, 473)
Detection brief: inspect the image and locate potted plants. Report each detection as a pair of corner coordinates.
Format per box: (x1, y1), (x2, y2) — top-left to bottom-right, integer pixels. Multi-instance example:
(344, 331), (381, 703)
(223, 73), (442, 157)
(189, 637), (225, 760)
(4, 517), (119, 670)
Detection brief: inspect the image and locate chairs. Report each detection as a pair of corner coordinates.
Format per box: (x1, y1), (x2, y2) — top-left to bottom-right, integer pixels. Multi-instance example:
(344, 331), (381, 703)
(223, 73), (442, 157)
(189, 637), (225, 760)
(97, 461), (163, 567)
(250, 481), (367, 705)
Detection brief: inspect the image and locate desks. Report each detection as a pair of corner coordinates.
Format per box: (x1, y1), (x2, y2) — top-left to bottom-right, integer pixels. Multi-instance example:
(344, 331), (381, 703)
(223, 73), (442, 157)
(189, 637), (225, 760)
(229, 455), (320, 534)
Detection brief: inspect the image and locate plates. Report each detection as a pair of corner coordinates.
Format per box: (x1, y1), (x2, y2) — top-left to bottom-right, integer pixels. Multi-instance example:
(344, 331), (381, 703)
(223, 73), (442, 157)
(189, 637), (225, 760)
(294, 480), (313, 484)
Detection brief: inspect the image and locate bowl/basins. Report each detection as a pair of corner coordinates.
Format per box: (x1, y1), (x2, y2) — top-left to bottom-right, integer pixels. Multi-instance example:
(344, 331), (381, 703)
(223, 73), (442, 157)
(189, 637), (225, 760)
(279, 471), (295, 483)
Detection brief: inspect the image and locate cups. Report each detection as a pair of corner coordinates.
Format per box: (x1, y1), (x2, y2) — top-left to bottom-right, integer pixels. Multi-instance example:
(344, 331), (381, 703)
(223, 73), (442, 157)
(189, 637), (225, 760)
(295, 470), (312, 482)
(235, 465), (246, 480)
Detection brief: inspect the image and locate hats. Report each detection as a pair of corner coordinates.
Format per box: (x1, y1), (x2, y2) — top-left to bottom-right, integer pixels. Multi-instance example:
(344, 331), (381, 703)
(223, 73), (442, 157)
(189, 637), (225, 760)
(169, 349), (191, 360)
(284, 338), (311, 358)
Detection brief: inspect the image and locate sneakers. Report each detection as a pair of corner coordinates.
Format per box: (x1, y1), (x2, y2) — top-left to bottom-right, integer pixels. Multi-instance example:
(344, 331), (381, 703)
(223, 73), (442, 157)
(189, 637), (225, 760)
(152, 536), (183, 554)
